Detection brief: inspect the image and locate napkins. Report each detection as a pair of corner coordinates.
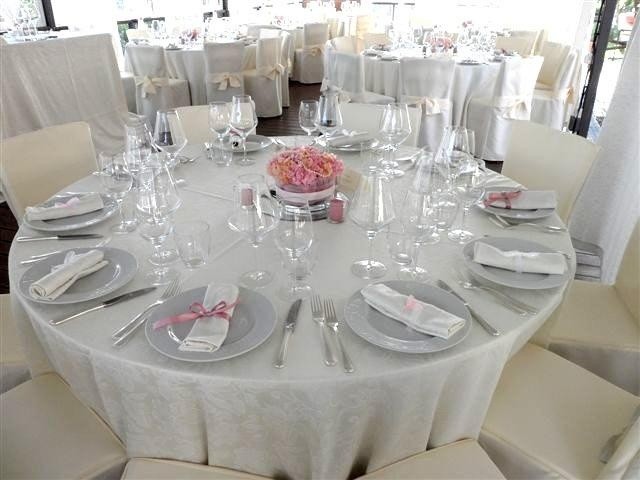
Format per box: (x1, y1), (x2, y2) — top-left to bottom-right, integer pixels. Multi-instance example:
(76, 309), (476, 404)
(358, 280), (468, 342)
(176, 281), (243, 357)
(484, 184), (558, 213)
(469, 241), (571, 276)
(29, 249), (112, 304)
(326, 129), (377, 149)
(24, 189), (106, 226)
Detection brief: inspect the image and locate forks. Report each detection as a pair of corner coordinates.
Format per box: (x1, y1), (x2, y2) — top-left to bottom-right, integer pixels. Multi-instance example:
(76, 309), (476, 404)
(310, 294), (355, 373)
(30, 238), (112, 261)
(112, 278), (183, 348)
(489, 213), (567, 236)
(450, 265), (538, 317)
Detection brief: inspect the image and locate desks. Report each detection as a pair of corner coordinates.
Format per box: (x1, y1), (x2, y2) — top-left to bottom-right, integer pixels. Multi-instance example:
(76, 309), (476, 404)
(7, 135), (577, 480)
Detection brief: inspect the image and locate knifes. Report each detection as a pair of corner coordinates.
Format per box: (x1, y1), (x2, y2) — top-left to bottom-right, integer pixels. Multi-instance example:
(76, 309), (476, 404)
(17, 234), (104, 243)
(48, 286), (157, 326)
(276, 298), (302, 368)
(438, 279), (500, 337)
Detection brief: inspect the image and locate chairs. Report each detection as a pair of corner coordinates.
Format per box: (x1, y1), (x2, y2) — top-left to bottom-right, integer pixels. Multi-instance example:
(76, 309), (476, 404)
(1, 119), (100, 226)
(528, 215), (640, 398)
(479, 341), (639, 479)
(499, 119), (603, 229)
(334, 103), (422, 148)
(162, 101), (260, 144)
(124, 16), (372, 128)
(119, 451), (274, 480)
(0, 371), (125, 479)
(343, 437), (506, 480)
(1, 291), (44, 386)
(316, 30), (583, 160)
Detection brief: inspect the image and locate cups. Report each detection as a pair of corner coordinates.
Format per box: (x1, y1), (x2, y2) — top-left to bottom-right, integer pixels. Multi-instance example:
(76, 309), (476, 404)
(137, 17), (275, 49)
(303, 0), (360, 17)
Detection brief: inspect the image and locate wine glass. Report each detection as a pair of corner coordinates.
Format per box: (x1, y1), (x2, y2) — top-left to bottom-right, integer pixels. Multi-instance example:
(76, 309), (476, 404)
(93, 93), (488, 297)
(385, 24), (497, 60)
(0, 2), (41, 45)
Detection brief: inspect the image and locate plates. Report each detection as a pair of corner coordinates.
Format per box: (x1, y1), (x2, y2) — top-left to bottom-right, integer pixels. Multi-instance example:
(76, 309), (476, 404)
(347, 281), (471, 355)
(462, 236), (570, 290)
(16, 245), (138, 305)
(145, 286), (276, 363)
(476, 186), (555, 220)
(23, 191), (119, 230)
